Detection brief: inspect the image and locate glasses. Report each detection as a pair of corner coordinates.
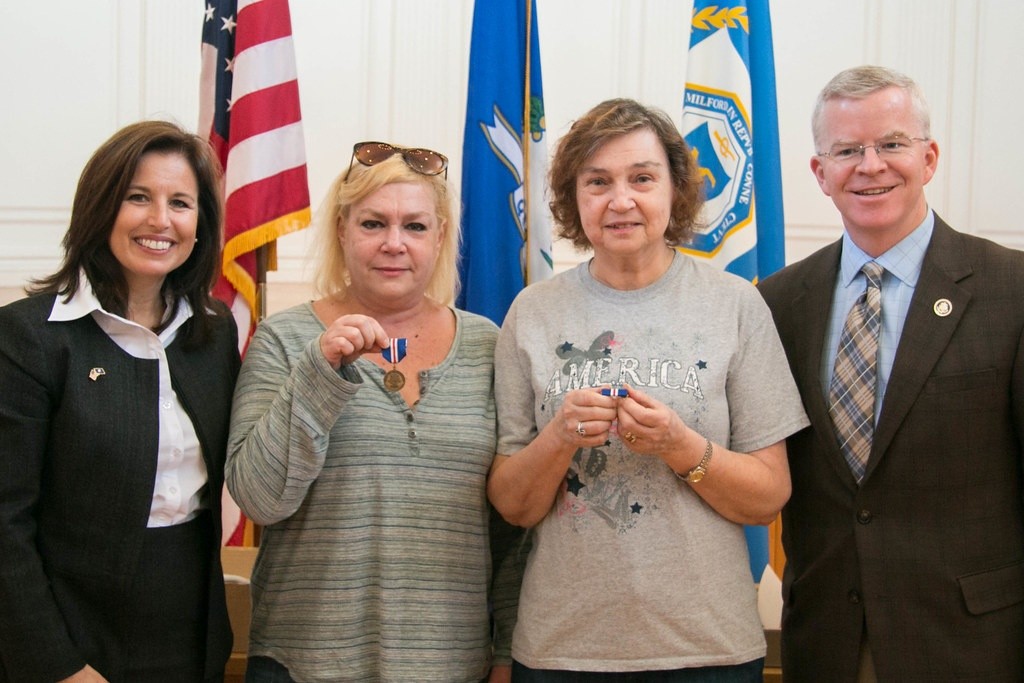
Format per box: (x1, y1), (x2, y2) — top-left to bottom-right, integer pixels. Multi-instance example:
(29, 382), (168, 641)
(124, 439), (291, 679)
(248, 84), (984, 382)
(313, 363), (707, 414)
(816, 134), (927, 168)
(343, 142), (449, 185)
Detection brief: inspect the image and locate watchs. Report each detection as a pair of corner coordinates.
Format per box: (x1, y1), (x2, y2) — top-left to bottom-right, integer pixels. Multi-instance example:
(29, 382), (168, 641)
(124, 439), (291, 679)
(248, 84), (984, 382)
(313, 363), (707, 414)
(675, 437), (713, 486)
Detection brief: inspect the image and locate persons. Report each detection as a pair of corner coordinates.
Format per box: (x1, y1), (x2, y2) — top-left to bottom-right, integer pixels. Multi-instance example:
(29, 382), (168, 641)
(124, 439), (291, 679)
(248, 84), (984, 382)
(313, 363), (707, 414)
(486, 95), (816, 683)
(223, 138), (510, 683)
(742, 65), (1024, 683)
(0, 120), (241, 683)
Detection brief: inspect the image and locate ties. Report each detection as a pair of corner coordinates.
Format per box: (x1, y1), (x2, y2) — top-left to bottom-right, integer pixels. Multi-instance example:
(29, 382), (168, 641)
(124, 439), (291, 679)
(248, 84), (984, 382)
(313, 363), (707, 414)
(827, 259), (886, 490)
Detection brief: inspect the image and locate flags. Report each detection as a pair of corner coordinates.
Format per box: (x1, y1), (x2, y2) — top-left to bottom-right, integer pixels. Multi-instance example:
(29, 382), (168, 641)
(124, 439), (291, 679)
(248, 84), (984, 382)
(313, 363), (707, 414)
(660, 0), (790, 633)
(192, 0), (312, 549)
(453, 1), (556, 596)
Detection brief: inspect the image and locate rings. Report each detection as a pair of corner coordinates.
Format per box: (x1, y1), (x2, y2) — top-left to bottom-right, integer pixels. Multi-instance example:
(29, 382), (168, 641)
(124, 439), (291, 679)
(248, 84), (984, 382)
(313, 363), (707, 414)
(575, 421), (587, 437)
(623, 431), (638, 447)
